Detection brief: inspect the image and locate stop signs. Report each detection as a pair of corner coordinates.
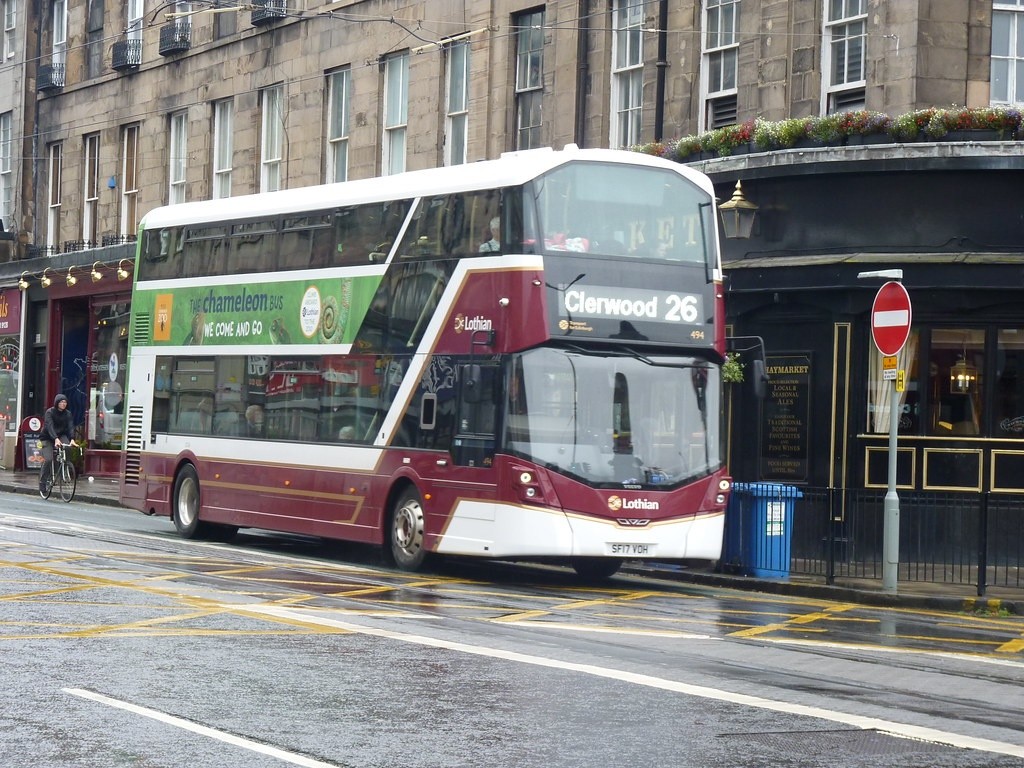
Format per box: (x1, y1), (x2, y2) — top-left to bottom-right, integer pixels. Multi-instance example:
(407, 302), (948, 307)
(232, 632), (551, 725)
(871, 279), (911, 357)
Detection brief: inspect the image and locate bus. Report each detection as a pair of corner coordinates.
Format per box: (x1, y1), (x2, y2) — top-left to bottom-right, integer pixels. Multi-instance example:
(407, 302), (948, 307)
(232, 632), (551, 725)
(119, 144), (772, 580)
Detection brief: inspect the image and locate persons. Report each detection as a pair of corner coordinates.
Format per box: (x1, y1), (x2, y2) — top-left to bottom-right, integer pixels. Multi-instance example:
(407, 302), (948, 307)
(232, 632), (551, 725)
(494, 371), (520, 433)
(339, 427), (355, 440)
(39, 394), (79, 492)
(238, 405), (266, 438)
(479, 217), (500, 252)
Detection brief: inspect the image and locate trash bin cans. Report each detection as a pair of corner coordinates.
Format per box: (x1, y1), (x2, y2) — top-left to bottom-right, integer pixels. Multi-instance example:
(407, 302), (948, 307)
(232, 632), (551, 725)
(718, 482), (802, 577)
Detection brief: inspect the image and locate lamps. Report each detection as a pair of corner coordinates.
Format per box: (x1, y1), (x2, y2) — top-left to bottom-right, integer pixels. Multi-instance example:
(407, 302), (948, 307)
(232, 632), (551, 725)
(950, 335), (979, 395)
(19, 271), (41, 292)
(713, 178), (758, 241)
(117, 259), (136, 281)
(66, 265), (90, 287)
(91, 261), (116, 284)
(41, 267), (65, 289)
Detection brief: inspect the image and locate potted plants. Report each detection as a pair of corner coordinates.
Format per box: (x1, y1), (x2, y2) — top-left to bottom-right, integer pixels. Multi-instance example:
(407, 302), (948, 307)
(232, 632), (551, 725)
(63, 440), (86, 480)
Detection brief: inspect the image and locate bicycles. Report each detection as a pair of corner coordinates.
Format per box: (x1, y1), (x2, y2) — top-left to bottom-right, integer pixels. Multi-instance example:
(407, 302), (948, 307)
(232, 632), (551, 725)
(38, 441), (83, 503)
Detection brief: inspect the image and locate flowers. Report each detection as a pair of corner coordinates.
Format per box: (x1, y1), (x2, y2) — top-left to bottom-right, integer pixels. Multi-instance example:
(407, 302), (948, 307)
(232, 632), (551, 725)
(721, 352), (746, 383)
(614, 101), (1024, 156)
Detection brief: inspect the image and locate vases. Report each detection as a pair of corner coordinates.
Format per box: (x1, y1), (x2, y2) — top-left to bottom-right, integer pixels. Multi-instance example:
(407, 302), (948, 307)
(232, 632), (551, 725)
(686, 128), (1024, 163)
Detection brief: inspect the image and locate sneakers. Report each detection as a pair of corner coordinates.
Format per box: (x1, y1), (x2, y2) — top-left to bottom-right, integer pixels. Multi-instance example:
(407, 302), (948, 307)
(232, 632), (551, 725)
(39, 481), (47, 493)
